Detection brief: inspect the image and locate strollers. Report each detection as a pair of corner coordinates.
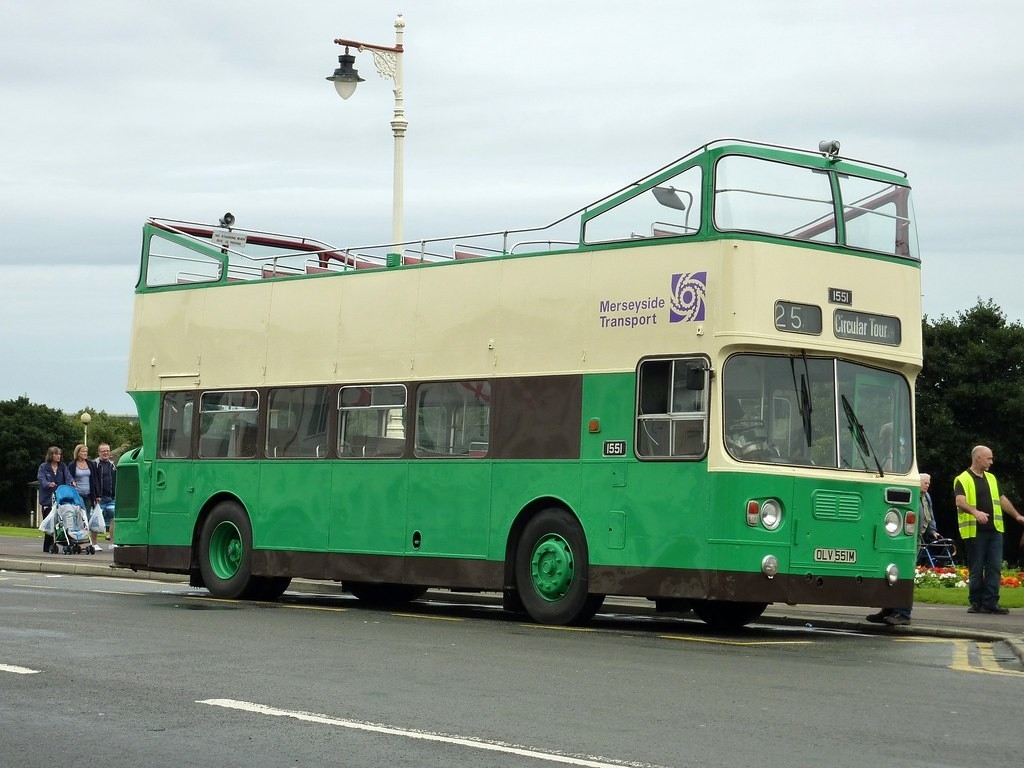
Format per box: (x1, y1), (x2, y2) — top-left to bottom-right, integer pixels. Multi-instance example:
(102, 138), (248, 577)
(49, 483), (96, 555)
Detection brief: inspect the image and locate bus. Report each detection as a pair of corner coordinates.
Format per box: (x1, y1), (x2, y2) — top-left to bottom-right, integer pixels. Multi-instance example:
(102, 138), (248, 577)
(107, 139), (922, 629)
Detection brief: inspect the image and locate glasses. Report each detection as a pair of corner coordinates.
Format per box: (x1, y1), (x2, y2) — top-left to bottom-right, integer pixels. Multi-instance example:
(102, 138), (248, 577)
(99, 450), (110, 453)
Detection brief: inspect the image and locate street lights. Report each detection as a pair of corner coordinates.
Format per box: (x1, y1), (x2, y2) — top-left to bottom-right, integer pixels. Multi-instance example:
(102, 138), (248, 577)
(81, 412), (92, 446)
(325, 12), (405, 265)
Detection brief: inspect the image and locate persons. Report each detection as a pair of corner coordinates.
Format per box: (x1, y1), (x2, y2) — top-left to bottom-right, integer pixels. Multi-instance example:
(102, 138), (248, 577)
(865, 422), (913, 625)
(954, 444), (1024, 615)
(722, 395), (773, 460)
(36, 441), (118, 552)
(916, 472), (943, 564)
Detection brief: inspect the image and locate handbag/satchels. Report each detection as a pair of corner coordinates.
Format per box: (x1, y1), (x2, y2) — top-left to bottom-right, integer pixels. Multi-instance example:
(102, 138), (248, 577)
(38, 503), (56, 536)
(87, 502), (107, 535)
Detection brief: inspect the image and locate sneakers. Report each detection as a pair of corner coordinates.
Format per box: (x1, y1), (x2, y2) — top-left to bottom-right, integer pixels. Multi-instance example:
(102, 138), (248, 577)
(866, 609), (895, 622)
(884, 610), (911, 626)
(108, 544), (118, 550)
(92, 544), (103, 551)
(967, 602), (982, 613)
(983, 604), (1009, 614)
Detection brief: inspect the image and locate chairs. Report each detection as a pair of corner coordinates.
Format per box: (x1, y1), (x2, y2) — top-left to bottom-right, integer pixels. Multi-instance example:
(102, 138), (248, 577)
(451, 243), (514, 259)
(175, 253), (386, 283)
(315, 436), (491, 458)
(402, 249), (453, 265)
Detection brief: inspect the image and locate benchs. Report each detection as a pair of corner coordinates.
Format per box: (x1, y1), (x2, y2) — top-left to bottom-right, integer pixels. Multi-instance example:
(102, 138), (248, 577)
(265, 441), (317, 458)
(650, 222), (699, 238)
